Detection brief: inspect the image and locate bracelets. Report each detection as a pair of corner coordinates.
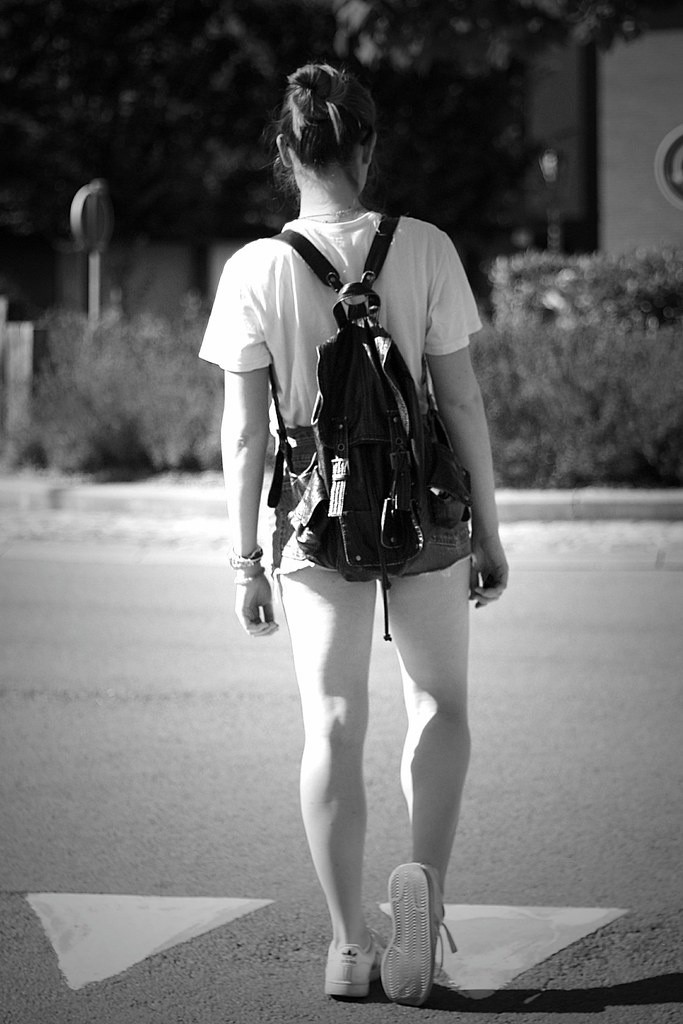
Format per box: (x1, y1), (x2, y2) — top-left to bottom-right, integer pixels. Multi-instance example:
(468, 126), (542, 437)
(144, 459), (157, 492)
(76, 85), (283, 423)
(234, 567), (266, 584)
(229, 545), (264, 569)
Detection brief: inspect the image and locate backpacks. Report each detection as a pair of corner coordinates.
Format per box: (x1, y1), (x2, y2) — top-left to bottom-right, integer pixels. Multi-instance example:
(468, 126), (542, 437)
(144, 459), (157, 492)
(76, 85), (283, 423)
(266, 212), (429, 586)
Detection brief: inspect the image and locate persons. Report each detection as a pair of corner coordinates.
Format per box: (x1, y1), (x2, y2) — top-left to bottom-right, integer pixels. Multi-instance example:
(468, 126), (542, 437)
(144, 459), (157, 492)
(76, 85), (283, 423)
(197, 65), (509, 1006)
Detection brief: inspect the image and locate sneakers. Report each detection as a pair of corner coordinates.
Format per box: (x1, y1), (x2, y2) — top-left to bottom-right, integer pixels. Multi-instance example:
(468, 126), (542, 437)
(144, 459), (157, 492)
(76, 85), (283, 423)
(324, 926), (386, 998)
(380, 863), (456, 1008)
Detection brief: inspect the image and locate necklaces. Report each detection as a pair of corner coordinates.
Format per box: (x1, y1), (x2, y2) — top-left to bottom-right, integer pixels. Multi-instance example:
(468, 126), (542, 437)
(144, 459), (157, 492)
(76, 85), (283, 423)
(298, 206), (363, 220)
